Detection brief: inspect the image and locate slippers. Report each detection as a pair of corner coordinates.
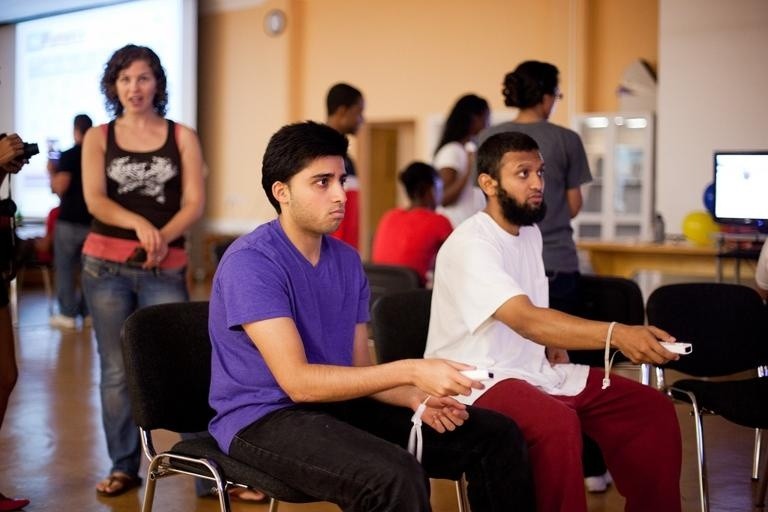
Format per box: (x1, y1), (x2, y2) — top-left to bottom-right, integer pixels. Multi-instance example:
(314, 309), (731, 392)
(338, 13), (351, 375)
(197, 485), (271, 504)
(95, 470), (143, 497)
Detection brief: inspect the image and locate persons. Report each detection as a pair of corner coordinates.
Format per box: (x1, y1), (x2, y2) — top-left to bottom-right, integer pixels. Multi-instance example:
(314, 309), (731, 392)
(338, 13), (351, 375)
(0, 127), (32, 510)
(753, 236), (767, 301)
(479, 60), (593, 275)
(431, 94), (490, 230)
(422, 132), (682, 510)
(206, 120), (541, 512)
(47, 114), (92, 331)
(369, 162), (454, 286)
(79, 45), (271, 505)
(320, 83), (364, 250)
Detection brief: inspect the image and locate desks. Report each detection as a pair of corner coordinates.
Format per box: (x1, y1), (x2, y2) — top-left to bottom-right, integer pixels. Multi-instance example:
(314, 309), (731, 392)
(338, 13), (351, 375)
(576, 239), (768, 295)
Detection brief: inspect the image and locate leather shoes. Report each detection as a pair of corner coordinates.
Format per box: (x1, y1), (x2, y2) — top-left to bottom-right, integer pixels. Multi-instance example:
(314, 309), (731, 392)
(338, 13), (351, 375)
(0, 493), (31, 511)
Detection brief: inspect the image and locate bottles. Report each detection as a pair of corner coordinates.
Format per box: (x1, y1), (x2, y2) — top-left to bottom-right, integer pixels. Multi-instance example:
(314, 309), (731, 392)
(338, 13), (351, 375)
(654, 216), (664, 241)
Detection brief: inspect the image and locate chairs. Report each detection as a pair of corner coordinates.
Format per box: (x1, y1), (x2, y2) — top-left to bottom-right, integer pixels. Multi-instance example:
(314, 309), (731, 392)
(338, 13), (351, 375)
(370, 289), (469, 512)
(363, 263), (420, 308)
(15, 207), (60, 316)
(549, 274), (665, 391)
(646, 283), (767, 511)
(121, 301), (326, 511)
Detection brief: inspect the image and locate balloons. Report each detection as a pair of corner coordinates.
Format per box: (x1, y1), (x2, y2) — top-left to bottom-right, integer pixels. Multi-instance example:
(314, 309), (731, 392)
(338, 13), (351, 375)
(682, 212), (720, 247)
(704, 184), (715, 215)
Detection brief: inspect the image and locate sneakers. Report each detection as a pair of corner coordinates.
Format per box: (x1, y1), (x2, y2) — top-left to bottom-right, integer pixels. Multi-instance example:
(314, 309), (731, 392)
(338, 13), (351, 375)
(83, 314), (93, 328)
(50, 314), (76, 329)
(584, 471), (614, 493)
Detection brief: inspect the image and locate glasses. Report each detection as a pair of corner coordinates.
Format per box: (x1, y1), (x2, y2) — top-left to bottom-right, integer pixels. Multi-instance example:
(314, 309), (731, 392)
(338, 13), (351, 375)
(550, 90), (565, 99)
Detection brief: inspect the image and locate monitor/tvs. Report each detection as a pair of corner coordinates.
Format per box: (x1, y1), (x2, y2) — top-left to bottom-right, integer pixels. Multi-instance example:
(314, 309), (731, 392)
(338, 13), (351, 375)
(713, 152), (768, 228)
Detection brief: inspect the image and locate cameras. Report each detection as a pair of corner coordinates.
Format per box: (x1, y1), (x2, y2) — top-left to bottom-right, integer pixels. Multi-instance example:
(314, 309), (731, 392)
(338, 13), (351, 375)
(0, 132), (40, 164)
